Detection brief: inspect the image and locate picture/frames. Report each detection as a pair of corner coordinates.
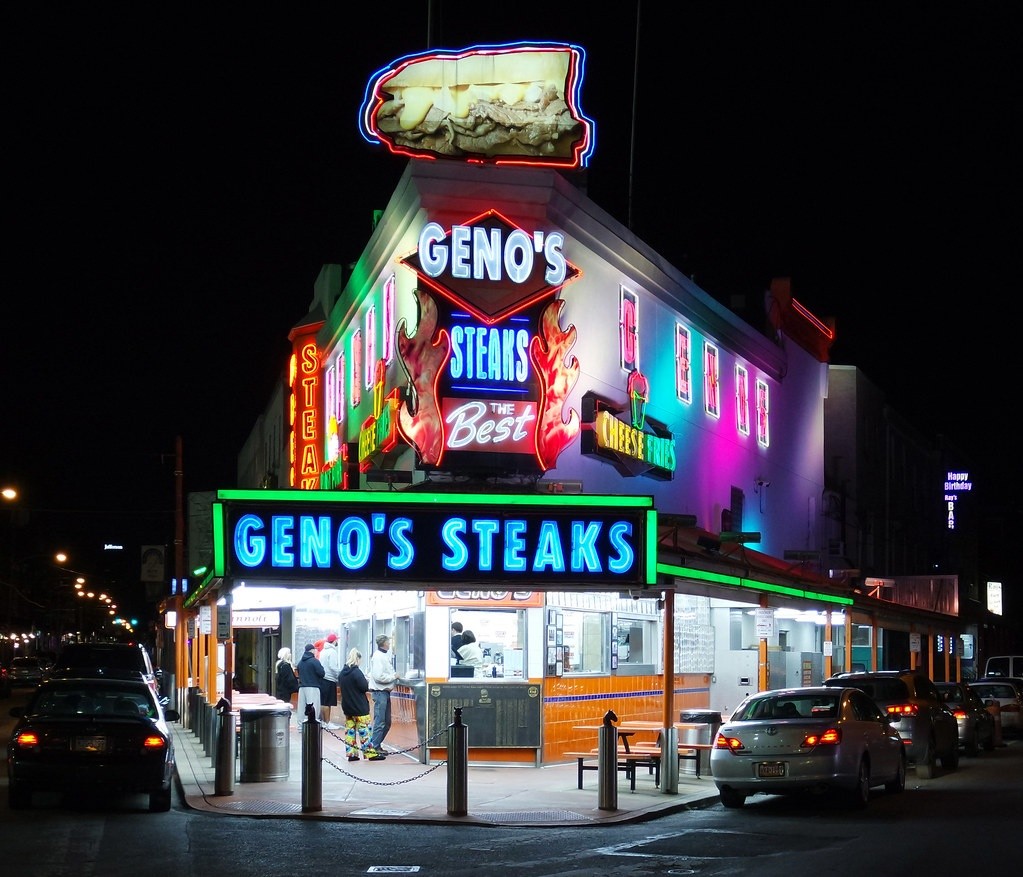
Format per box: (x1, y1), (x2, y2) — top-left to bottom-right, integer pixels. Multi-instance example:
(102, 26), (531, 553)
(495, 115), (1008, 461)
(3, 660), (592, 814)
(547, 610), (563, 677)
(610, 612), (618, 670)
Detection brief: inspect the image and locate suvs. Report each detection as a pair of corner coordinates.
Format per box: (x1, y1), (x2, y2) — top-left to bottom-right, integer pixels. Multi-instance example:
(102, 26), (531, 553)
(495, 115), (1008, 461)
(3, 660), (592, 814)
(981, 677), (1023, 702)
(824, 668), (959, 779)
(40, 637), (163, 696)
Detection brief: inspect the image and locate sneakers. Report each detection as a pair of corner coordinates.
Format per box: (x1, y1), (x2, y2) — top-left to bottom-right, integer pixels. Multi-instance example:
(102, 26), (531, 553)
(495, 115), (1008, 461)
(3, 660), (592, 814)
(321, 720), (327, 729)
(325, 721), (340, 730)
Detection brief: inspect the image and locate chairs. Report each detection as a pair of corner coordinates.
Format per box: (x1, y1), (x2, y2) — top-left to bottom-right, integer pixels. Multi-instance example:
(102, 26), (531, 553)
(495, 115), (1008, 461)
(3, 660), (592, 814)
(780, 702), (802, 718)
(61, 695), (95, 714)
(112, 698), (141, 714)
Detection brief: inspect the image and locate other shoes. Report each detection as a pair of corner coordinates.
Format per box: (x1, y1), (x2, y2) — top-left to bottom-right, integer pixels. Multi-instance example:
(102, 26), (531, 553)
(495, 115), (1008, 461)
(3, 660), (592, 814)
(347, 756), (360, 761)
(369, 755), (386, 760)
(376, 747), (388, 753)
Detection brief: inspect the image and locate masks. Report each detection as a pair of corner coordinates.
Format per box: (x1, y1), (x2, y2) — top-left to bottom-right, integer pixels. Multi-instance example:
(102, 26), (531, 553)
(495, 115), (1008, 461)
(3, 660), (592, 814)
(335, 641), (338, 647)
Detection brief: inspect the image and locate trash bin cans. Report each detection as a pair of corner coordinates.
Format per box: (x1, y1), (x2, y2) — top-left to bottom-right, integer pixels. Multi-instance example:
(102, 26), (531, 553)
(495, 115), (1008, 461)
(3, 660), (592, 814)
(239, 708), (293, 782)
(677, 710), (722, 776)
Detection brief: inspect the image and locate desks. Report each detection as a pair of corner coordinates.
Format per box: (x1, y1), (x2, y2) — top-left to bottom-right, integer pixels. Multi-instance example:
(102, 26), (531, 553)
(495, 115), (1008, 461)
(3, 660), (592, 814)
(620, 720), (709, 729)
(571, 726), (662, 753)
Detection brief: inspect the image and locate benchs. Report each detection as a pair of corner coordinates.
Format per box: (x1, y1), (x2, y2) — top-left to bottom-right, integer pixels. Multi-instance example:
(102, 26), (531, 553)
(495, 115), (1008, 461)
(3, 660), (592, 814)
(563, 742), (712, 795)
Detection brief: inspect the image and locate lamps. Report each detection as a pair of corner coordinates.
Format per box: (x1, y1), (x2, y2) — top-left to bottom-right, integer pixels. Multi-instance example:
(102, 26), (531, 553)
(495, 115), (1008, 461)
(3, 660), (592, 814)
(829, 569), (860, 585)
(864, 578), (896, 597)
(656, 513), (697, 554)
(782, 550), (819, 582)
(712, 532), (761, 568)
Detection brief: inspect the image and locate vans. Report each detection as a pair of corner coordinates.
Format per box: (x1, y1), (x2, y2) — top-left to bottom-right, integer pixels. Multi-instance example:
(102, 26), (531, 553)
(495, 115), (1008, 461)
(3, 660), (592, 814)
(984, 655), (1023, 681)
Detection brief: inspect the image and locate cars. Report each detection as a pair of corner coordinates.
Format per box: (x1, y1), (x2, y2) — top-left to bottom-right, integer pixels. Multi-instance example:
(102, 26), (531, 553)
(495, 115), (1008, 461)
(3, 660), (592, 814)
(964, 682), (1023, 741)
(933, 682), (995, 757)
(710, 687), (907, 812)
(0, 655), (46, 698)
(5, 666), (182, 813)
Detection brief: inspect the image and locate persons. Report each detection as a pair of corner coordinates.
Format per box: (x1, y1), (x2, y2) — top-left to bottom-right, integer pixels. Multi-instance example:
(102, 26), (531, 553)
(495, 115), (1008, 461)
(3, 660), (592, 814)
(455, 630), (484, 678)
(451, 622), (463, 664)
(319, 634), (342, 730)
(367, 634), (399, 756)
(338, 648), (387, 761)
(297, 644), (324, 731)
(275, 647), (298, 703)
(309, 640), (324, 658)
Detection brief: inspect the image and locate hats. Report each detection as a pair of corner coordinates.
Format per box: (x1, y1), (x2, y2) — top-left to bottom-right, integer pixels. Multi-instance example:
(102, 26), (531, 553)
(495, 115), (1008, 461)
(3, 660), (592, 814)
(375, 634), (394, 645)
(328, 634), (340, 643)
(305, 643), (317, 651)
